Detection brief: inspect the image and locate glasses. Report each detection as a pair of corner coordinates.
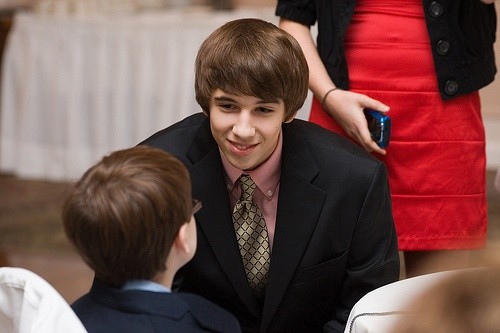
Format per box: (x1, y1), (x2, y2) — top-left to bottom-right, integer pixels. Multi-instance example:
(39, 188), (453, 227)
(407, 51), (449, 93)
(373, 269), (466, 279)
(192, 198), (203, 218)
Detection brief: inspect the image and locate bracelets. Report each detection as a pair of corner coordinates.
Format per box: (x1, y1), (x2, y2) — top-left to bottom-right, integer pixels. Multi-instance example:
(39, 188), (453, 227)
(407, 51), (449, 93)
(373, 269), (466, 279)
(321, 88), (338, 112)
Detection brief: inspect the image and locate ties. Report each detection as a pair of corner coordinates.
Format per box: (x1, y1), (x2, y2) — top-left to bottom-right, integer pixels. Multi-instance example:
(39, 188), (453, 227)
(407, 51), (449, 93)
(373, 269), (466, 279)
(232, 175), (272, 311)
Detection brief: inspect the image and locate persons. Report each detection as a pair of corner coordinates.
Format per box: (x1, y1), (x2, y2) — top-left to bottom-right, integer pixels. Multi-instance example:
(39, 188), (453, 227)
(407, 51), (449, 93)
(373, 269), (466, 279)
(55, 144), (241, 332)
(126, 18), (401, 333)
(273, 0), (498, 280)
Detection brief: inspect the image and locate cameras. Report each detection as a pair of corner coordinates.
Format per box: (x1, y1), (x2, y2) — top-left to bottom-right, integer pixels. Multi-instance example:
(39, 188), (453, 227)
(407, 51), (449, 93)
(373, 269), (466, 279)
(364, 107), (391, 148)
